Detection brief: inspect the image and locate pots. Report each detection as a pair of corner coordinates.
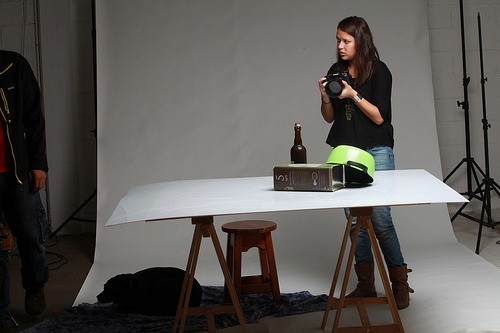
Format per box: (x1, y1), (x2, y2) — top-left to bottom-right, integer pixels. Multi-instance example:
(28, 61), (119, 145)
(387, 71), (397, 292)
(328, 144), (376, 184)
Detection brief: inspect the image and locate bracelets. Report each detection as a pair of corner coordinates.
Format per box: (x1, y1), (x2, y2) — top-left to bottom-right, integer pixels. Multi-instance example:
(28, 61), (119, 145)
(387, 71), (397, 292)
(353, 93), (362, 103)
(322, 101), (331, 104)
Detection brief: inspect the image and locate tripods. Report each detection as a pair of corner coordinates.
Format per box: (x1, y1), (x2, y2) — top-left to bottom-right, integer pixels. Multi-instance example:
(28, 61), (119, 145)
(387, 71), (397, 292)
(445, 0), (499, 256)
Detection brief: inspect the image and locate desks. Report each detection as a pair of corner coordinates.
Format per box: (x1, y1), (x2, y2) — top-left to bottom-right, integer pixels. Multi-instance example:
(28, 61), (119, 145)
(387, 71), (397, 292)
(102, 166), (470, 333)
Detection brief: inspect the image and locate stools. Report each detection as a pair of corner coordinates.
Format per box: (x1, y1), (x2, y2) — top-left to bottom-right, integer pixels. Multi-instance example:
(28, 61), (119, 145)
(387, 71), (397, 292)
(221, 219), (281, 301)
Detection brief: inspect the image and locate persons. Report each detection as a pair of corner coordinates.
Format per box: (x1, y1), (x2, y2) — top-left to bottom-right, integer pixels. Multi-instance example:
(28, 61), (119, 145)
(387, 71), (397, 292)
(0, 50), (49, 316)
(318, 16), (414, 309)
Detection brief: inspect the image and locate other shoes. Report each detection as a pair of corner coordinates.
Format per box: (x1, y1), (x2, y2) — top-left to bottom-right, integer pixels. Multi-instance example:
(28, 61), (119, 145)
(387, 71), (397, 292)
(25, 286), (47, 313)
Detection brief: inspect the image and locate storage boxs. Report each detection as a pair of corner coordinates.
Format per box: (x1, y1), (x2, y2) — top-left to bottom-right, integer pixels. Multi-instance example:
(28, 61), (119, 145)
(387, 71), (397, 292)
(272, 162), (346, 192)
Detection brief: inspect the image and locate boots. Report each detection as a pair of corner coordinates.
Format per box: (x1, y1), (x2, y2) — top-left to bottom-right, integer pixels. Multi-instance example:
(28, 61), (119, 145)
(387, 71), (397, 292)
(387, 264), (414, 310)
(344, 261), (375, 299)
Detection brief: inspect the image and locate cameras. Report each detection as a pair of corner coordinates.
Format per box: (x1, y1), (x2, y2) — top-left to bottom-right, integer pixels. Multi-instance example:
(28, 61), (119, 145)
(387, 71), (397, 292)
(323, 72), (354, 96)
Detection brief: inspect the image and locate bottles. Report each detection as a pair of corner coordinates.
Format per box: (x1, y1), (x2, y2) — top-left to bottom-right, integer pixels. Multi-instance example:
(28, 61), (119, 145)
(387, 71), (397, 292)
(290, 121), (308, 163)
